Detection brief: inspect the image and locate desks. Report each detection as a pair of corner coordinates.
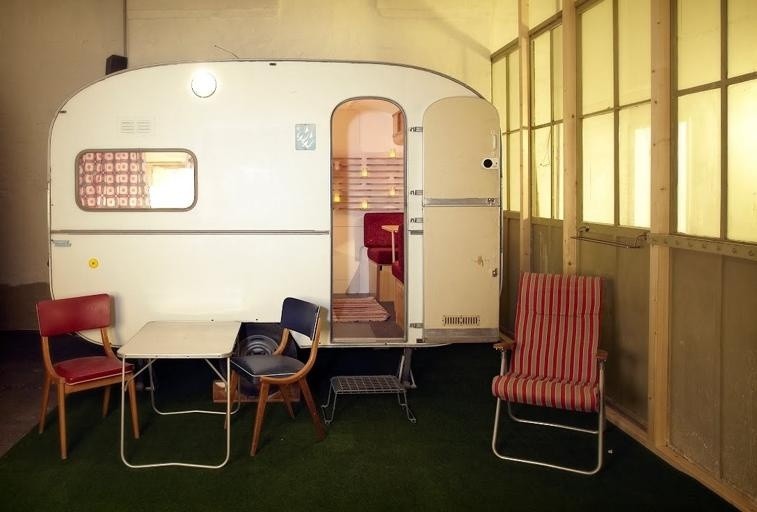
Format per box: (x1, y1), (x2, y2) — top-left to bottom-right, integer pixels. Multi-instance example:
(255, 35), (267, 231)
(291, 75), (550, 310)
(116, 320), (243, 470)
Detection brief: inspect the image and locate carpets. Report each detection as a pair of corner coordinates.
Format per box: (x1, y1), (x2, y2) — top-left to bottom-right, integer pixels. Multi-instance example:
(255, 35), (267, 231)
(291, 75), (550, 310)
(331, 296), (390, 323)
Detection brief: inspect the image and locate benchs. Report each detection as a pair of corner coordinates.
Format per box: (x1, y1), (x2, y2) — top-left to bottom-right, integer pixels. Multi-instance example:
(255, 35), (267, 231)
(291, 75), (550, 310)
(362, 211), (404, 329)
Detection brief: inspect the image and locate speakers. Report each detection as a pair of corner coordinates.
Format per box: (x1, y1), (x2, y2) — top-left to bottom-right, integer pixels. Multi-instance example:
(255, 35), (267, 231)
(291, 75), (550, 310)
(105, 54), (127, 74)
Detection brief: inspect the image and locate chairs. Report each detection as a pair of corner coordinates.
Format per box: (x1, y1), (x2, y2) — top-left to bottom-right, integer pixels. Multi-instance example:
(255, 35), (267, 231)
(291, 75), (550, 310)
(35, 294), (140, 460)
(491, 272), (608, 476)
(224, 296), (325, 456)
